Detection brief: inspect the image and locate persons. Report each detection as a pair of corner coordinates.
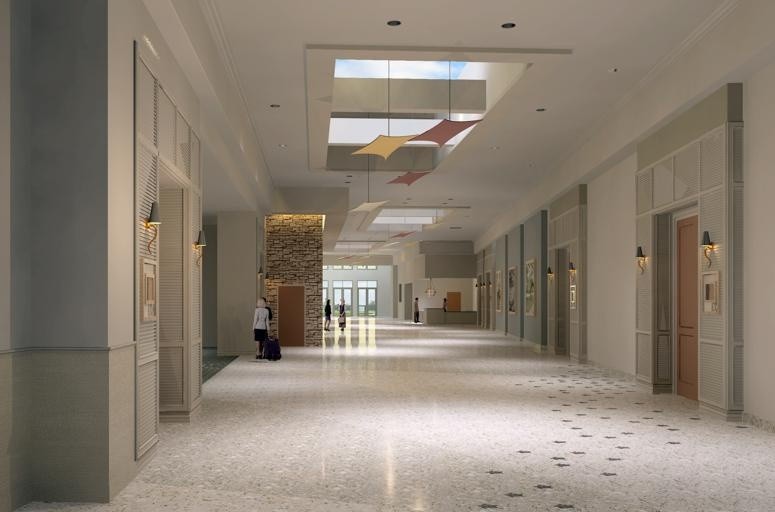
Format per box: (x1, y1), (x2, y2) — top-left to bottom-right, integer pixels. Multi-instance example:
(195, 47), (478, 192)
(413, 297), (420, 323)
(339, 299), (346, 331)
(324, 299), (331, 331)
(262, 297), (273, 320)
(252, 298), (271, 360)
(442, 297), (448, 312)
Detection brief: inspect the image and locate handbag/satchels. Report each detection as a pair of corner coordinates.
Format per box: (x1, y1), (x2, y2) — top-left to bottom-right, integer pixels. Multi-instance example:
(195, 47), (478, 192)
(338, 317), (344, 323)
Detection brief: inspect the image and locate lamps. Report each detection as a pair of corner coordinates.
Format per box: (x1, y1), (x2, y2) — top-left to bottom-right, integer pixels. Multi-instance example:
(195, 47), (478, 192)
(634, 245), (648, 274)
(546, 262), (576, 281)
(192, 230), (206, 267)
(699, 231), (714, 268)
(145, 200), (161, 254)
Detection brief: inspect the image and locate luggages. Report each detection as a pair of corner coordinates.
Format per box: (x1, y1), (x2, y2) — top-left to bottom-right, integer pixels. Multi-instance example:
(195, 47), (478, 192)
(263, 336), (281, 361)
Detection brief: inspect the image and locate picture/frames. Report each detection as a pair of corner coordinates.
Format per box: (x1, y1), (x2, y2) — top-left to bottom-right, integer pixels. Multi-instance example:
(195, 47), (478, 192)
(494, 259), (535, 316)
